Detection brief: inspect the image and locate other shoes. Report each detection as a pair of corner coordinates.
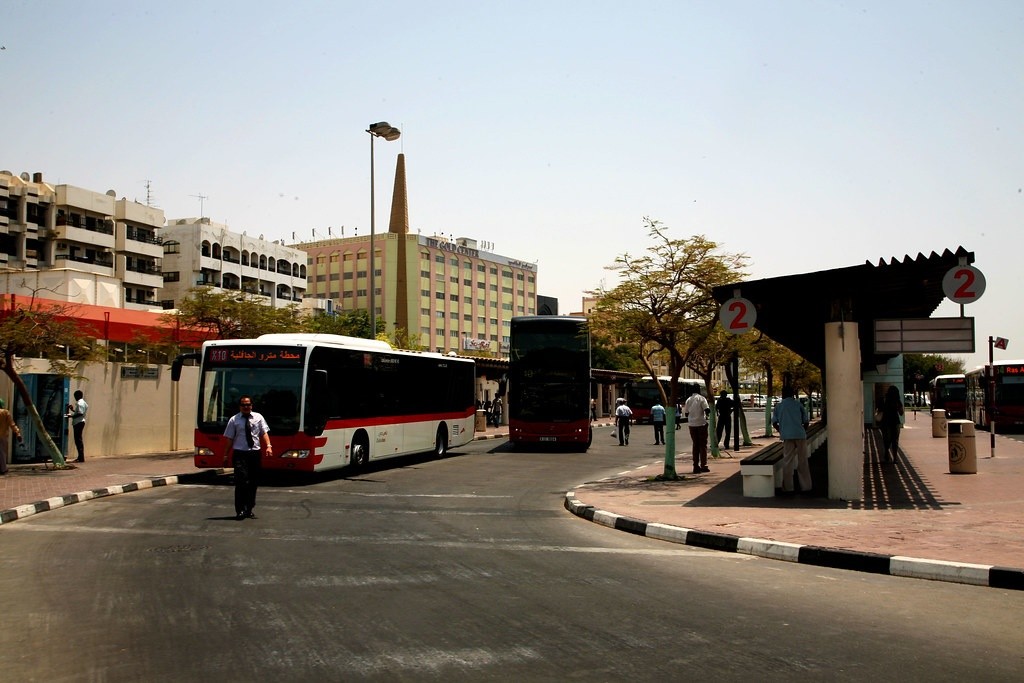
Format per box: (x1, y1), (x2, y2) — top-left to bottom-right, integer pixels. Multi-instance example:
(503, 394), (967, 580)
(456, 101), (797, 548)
(679, 426), (681, 429)
(662, 440), (665, 445)
(701, 468), (710, 472)
(653, 442), (659, 445)
(73, 458), (85, 463)
(619, 443), (624, 446)
(625, 437), (628, 446)
(693, 468), (700, 473)
(676, 427), (679, 430)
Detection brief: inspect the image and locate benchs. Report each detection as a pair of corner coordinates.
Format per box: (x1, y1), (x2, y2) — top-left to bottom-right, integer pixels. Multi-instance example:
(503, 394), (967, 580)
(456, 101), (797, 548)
(739, 420), (826, 497)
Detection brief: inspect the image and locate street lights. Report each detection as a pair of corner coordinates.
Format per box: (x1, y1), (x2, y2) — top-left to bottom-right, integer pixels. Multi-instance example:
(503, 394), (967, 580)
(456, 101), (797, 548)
(366, 121), (401, 337)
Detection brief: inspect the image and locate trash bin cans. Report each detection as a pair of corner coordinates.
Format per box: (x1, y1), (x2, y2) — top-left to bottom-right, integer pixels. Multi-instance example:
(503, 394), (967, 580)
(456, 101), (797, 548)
(948, 419), (978, 475)
(476, 410), (486, 432)
(932, 409), (948, 438)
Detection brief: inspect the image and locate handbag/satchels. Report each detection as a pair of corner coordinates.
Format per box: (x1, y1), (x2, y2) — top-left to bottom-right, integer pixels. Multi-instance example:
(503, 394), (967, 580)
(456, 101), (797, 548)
(611, 431), (617, 438)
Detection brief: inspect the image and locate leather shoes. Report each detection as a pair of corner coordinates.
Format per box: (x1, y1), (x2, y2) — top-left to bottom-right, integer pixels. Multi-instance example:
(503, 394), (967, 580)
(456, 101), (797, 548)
(245, 512), (254, 518)
(236, 511), (246, 520)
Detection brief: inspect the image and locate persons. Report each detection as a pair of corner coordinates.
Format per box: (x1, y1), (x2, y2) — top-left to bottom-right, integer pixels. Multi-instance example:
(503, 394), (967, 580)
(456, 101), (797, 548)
(222, 395), (272, 520)
(676, 399), (682, 429)
(614, 395), (633, 446)
(881, 385), (904, 464)
(476, 393), (504, 428)
(709, 390), (735, 449)
(651, 398), (665, 445)
(66, 390), (88, 463)
(591, 396), (598, 421)
(0, 399), (22, 475)
(772, 387), (815, 495)
(684, 383), (711, 474)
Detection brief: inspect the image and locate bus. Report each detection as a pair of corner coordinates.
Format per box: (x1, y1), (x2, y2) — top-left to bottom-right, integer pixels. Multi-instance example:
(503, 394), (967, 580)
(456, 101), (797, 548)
(929, 374), (965, 417)
(624, 376), (707, 424)
(965, 359), (1024, 429)
(507, 315), (597, 448)
(194, 334), (474, 471)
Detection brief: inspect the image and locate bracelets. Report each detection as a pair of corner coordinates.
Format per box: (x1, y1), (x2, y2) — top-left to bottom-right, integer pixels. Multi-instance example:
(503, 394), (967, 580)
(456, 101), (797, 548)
(223, 455), (228, 457)
(266, 444), (272, 447)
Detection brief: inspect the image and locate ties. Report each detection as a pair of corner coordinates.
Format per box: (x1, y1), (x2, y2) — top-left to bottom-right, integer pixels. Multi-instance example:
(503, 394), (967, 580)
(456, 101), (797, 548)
(242, 415), (254, 448)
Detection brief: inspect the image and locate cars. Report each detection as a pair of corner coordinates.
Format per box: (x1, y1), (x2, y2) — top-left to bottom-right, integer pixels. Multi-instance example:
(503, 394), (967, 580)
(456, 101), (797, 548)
(714, 396), (720, 404)
(754, 395), (782, 406)
(904, 394), (930, 407)
(741, 398), (760, 407)
